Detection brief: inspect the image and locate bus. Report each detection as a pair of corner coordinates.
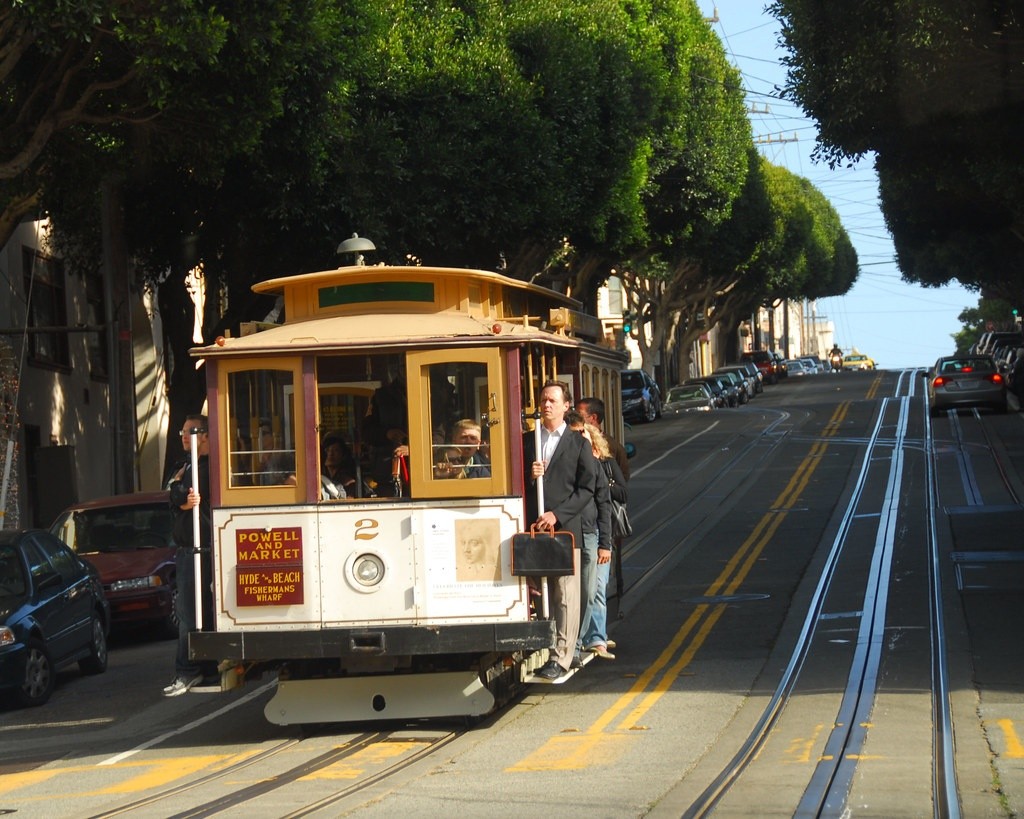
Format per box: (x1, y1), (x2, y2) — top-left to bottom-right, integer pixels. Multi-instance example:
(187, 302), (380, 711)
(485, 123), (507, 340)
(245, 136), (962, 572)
(166, 233), (638, 731)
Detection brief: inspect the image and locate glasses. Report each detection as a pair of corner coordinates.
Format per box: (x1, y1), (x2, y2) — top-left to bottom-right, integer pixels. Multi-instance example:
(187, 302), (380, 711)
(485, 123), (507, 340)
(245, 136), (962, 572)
(178, 427), (207, 436)
(260, 429), (271, 435)
(573, 430), (584, 435)
(449, 457), (465, 465)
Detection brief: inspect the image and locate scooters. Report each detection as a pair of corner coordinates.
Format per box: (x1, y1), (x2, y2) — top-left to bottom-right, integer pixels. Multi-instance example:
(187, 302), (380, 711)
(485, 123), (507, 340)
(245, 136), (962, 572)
(829, 355), (843, 373)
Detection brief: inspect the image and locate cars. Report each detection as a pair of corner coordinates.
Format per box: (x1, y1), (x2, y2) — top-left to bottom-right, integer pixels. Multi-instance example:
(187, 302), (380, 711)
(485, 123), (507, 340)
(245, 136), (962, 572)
(0, 525), (112, 709)
(921, 354), (1008, 417)
(708, 373), (741, 408)
(842, 354), (879, 371)
(968, 330), (1024, 398)
(774, 353), (830, 376)
(720, 364), (755, 404)
(620, 369), (664, 423)
(51, 490), (187, 635)
(662, 378), (730, 416)
(737, 361), (763, 393)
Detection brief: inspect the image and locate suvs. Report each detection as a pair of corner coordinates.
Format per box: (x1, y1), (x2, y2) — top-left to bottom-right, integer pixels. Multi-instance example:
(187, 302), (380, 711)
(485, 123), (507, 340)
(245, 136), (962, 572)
(743, 349), (779, 385)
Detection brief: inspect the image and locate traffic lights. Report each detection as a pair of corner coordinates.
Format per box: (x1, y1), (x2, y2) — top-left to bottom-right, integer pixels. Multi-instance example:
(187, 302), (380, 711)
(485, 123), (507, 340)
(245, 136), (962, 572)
(1012, 309), (1019, 316)
(622, 310), (632, 333)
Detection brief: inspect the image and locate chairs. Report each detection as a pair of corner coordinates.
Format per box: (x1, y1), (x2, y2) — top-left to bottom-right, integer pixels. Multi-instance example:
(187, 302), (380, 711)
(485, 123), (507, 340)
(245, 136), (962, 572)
(150, 513), (172, 539)
(944, 364), (957, 372)
(629, 376), (641, 388)
(975, 362), (990, 371)
(87, 524), (121, 547)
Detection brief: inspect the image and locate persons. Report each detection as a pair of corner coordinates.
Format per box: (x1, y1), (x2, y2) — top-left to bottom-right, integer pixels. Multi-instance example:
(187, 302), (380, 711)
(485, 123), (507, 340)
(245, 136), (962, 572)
(161, 415), (221, 698)
(829, 344), (843, 369)
(239, 352), (491, 501)
(522, 380), (631, 679)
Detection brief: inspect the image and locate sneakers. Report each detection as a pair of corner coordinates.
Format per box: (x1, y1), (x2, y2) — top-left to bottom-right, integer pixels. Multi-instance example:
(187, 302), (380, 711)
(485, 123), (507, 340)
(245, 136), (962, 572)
(160, 667), (203, 698)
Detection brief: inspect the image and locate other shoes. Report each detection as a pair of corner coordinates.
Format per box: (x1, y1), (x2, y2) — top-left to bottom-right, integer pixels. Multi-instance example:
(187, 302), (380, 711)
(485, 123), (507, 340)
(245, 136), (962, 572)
(569, 657), (584, 668)
(583, 641), (617, 651)
(588, 646), (616, 661)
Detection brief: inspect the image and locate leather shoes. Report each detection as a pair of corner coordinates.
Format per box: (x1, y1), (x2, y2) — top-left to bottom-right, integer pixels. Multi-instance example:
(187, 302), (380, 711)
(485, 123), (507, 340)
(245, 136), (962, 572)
(533, 661), (563, 680)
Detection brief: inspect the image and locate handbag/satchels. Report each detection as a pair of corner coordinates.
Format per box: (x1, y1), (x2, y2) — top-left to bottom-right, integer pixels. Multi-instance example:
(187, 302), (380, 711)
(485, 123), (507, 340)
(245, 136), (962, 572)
(510, 523), (575, 577)
(604, 461), (633, 539)
